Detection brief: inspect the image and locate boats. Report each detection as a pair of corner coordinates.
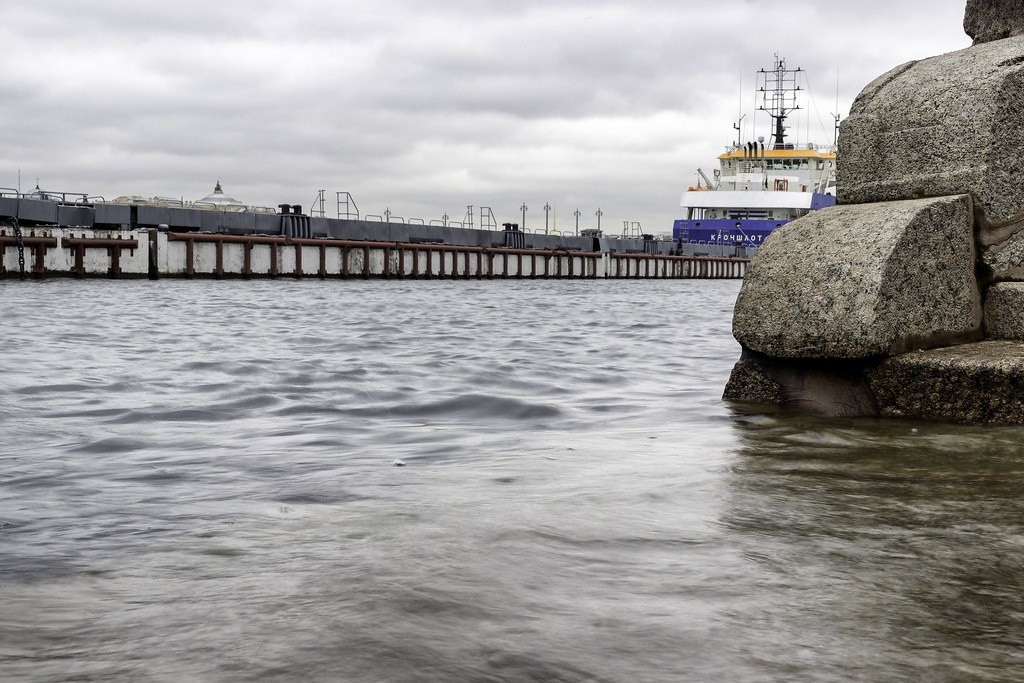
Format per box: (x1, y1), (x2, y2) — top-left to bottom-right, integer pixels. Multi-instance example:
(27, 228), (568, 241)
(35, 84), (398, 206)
(672, 49), (841, 250)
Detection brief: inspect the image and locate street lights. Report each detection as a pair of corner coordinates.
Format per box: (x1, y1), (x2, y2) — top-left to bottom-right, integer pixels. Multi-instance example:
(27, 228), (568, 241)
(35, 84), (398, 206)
(595, 207), (603, 231)
(442, 212), (449, 228)
(543, 201), (552, 234)
(520, 202), (528, 233)
(573, 208), (581, 236)
